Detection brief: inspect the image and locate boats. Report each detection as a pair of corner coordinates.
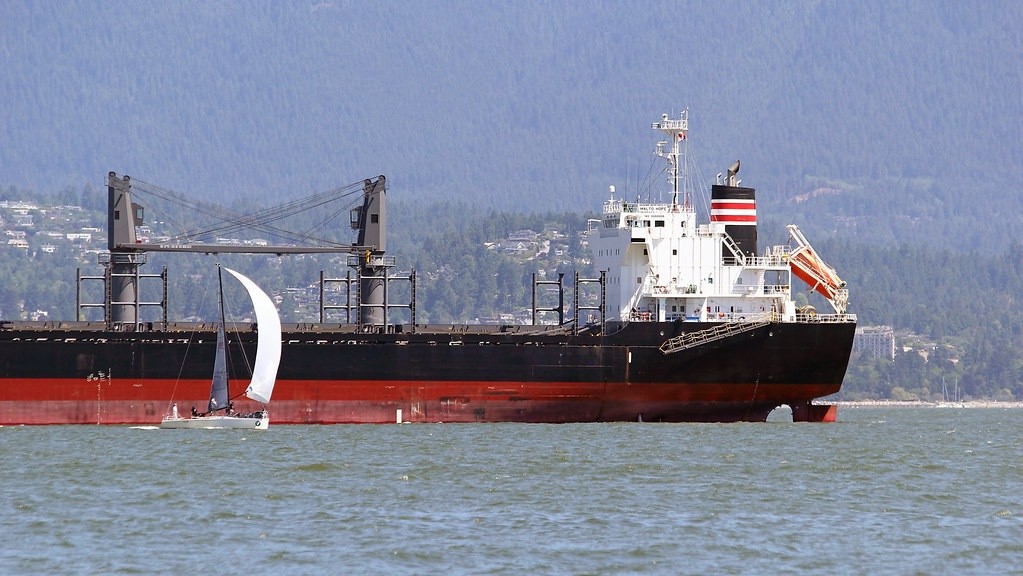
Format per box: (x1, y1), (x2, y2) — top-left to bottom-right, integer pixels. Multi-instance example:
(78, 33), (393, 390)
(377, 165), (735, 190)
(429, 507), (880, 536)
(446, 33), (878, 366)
(0, 104), (858, 426)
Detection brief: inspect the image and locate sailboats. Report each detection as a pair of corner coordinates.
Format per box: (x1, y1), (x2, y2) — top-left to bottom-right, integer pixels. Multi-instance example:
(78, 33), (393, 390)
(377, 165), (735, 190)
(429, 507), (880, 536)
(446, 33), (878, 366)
(159, 262), (284, 432)
